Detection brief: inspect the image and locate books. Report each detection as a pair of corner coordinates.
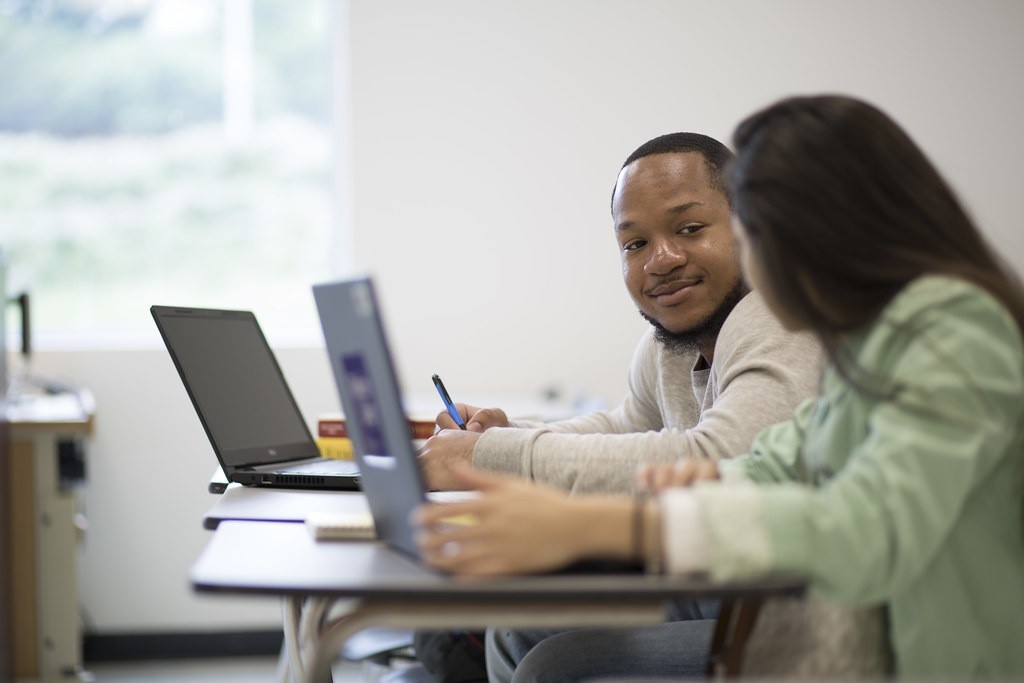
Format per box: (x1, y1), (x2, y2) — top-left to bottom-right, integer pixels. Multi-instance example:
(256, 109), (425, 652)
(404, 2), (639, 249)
(311, 411), (438, 461)
(302, 509), (381, 543)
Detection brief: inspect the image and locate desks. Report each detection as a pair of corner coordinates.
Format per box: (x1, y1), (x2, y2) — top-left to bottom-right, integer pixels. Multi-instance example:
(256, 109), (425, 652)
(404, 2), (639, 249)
(187, 460), (808, 683)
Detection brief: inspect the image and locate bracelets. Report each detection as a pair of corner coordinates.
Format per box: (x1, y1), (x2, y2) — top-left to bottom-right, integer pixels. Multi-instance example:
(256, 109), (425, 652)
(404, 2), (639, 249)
(627, 492), (649, 566)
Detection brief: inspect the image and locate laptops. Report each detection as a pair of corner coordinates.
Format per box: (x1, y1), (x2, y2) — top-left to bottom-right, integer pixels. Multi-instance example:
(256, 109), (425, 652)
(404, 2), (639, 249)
(311, 279), (427, 560)
(151, 305), (361, 493)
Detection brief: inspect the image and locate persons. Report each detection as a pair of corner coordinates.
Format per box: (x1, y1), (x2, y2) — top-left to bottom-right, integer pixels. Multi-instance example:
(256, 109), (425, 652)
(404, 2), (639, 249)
(413, 131), (894, 683)
(406, 95), (1024, 683)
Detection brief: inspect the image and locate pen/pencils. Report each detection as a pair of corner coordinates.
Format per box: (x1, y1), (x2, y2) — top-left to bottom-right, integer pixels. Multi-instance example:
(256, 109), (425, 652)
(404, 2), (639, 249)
(431, 374), (467, 431)
